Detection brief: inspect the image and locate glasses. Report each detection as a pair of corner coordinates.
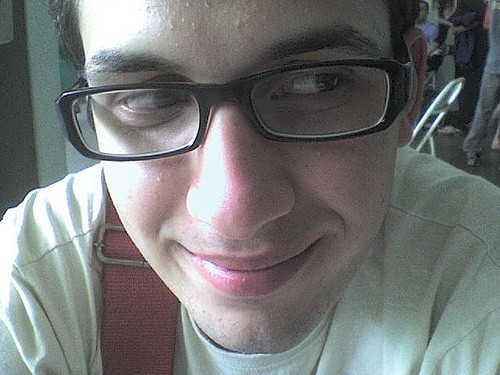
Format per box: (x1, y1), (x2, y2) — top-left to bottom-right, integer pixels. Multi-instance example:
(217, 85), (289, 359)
(54, 58), (415, 162)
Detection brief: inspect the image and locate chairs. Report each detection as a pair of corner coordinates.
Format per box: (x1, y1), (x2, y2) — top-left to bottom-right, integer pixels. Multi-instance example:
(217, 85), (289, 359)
(406, 78), (466, 156)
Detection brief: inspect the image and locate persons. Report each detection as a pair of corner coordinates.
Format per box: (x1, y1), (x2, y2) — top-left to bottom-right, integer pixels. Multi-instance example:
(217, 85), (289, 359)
(0, 0), (500, 375)
(416, 0), (500, 167)
